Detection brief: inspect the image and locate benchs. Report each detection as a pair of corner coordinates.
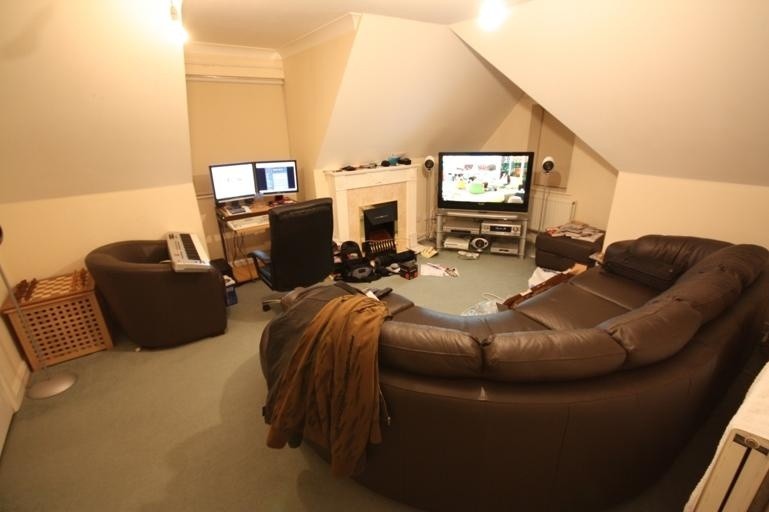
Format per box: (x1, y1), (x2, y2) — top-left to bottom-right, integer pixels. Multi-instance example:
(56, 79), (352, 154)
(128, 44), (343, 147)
(535, 227), (606, 272)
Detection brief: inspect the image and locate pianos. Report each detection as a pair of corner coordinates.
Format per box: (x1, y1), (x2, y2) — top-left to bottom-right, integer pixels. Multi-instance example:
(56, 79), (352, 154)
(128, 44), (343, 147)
(166, 231), (210, 274)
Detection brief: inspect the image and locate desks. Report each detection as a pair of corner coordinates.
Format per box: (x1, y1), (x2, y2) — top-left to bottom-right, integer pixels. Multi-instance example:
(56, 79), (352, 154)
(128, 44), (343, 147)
(210, 197), (298, 286)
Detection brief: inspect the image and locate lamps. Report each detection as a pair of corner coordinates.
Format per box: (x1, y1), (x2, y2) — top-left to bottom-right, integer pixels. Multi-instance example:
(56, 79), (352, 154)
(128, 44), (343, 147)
(528, 156), (555, 259)
(419, 155), (436, 249)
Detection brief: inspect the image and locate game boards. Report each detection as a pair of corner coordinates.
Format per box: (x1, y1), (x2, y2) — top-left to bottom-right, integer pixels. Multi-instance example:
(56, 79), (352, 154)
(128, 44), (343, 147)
(14, 267), (87, 303)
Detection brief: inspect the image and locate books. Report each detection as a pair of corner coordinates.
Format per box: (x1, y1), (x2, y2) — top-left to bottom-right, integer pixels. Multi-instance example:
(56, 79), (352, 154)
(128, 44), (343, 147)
(546, 226), (604, 243)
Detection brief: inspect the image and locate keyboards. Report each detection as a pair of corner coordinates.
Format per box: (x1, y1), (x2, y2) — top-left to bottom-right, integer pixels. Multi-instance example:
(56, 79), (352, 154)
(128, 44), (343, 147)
(227, 214), (270, 231)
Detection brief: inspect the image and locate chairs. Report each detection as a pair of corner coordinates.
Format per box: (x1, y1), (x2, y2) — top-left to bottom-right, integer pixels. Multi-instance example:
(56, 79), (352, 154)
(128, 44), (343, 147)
(248, 196), (333, 313)
(84, 238), (227, 350)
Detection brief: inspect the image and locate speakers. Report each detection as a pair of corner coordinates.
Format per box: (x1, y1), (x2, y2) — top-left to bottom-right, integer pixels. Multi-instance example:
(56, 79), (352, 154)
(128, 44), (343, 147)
(542, 156), (555, 173)
(424, 156), (435, 172)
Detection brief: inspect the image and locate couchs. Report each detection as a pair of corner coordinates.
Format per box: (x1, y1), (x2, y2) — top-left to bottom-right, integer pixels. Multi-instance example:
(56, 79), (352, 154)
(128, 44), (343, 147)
(258, 233), (769, 512)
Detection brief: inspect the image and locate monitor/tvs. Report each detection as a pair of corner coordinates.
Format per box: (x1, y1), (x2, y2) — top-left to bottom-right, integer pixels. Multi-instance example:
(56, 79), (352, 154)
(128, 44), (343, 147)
(208, 162), (258, 214)
(254, 160), (299, 207)
(438, 151), (534, 221)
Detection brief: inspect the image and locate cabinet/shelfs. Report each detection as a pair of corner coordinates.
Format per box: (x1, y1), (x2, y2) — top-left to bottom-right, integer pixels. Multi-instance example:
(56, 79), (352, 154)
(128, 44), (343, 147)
(432, 206), (529, 259)
(1, 269), (113, 371)
